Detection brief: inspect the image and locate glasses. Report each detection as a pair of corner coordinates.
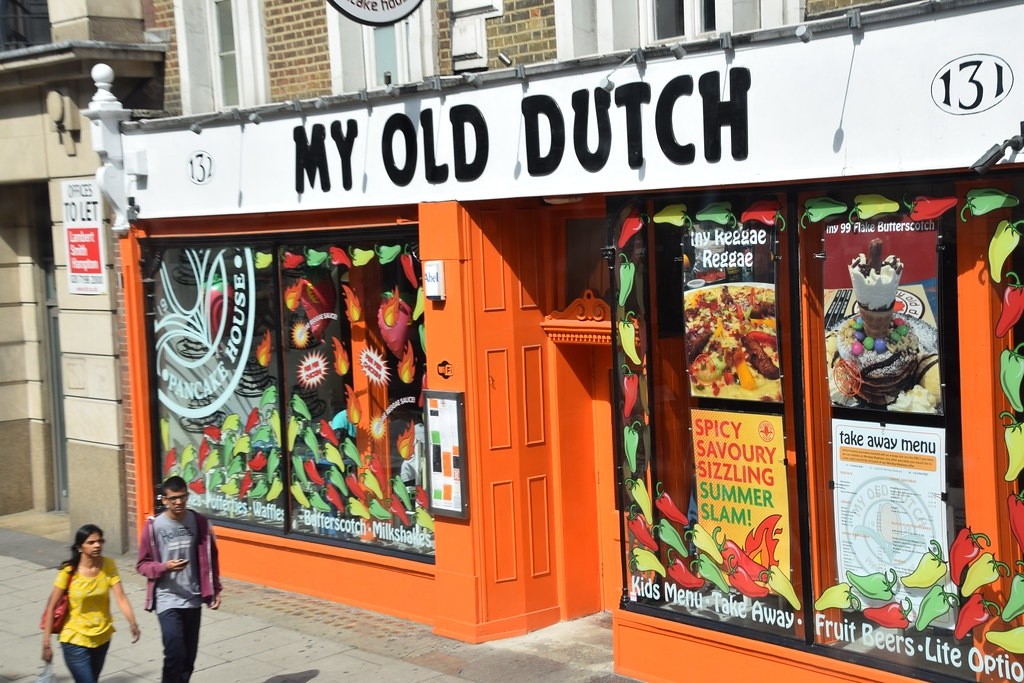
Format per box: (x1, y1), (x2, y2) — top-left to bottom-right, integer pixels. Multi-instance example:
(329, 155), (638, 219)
(164, 492), (190, 504)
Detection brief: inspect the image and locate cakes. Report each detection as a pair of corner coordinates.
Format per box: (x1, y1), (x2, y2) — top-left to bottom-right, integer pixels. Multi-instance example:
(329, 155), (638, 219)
(835, 237), (919, 406)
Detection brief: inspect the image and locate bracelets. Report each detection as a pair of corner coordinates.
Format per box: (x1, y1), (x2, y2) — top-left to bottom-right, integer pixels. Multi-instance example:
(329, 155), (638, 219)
(44, 646), (49, 649)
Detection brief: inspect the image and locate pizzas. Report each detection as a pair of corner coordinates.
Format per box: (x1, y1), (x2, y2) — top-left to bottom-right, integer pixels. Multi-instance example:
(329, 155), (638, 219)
(683, 285), (784, 403)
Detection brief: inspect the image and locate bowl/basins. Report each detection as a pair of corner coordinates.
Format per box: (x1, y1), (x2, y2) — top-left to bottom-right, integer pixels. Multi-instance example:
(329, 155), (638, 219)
(687, 278), (705, 288)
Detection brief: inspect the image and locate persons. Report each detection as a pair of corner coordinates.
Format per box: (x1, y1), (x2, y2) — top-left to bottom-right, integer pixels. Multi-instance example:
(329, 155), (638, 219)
(43, 525), (140, 683)
(137, 476), (222, 683)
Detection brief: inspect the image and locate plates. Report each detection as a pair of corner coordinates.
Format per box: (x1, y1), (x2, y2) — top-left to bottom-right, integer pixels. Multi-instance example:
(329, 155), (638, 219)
(683, 282), (784, 403)
(825, 310), (942, 417)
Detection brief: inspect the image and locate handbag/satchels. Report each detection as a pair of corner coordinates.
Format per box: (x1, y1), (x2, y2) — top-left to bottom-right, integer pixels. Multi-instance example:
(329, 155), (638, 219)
(40, 565), (76, 633)
